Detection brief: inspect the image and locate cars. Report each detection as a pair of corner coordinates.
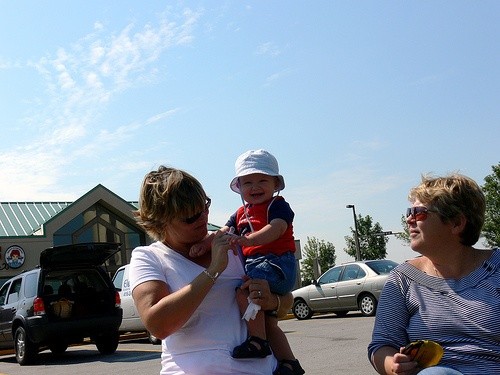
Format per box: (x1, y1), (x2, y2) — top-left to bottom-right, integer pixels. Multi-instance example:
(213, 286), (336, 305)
(290, 260), (399, 320)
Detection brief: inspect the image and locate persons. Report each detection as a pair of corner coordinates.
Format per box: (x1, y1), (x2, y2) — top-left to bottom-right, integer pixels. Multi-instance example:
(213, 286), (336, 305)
(188, 149), (305, 375)
(130, 166), (294, 375)
(367, 169), (500, 375)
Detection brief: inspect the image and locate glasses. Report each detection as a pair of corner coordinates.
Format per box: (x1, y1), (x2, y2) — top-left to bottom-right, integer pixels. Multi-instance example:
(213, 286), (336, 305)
(172, 196), (211, 224)
(406, 207), (450, 221)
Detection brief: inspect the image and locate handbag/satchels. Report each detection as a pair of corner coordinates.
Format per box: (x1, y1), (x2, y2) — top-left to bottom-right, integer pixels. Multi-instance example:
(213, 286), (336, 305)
(53, 298), (73, 318)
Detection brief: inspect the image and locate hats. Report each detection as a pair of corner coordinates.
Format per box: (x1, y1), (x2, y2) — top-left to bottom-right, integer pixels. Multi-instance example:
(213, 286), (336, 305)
(229, 150), (285, 194)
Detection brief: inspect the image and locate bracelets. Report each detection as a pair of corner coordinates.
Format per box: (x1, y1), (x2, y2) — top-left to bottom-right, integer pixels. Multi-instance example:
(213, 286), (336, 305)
(203, 270), (216, 284)
(276, 295), (281, 312)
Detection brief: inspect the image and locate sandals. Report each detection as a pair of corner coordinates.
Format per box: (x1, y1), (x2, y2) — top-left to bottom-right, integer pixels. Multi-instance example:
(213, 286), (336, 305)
(233, 335), (272, 359)
(272, 358), (306, 375)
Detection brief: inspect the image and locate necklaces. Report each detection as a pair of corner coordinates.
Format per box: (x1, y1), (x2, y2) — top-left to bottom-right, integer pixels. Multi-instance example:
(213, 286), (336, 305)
(164, 240), (184, 257)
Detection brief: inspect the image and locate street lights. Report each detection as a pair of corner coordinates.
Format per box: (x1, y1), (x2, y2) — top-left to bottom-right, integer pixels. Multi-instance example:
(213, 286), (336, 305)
(346, 204), (362, 261)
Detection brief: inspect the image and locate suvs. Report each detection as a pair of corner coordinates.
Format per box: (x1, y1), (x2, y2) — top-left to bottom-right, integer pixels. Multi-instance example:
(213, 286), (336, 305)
(113, 263), (162, 344)
(0, 242), (123, 364)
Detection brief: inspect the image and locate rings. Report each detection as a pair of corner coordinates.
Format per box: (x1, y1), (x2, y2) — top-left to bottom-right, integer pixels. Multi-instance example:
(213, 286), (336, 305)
(258, 291), (261, 295)
